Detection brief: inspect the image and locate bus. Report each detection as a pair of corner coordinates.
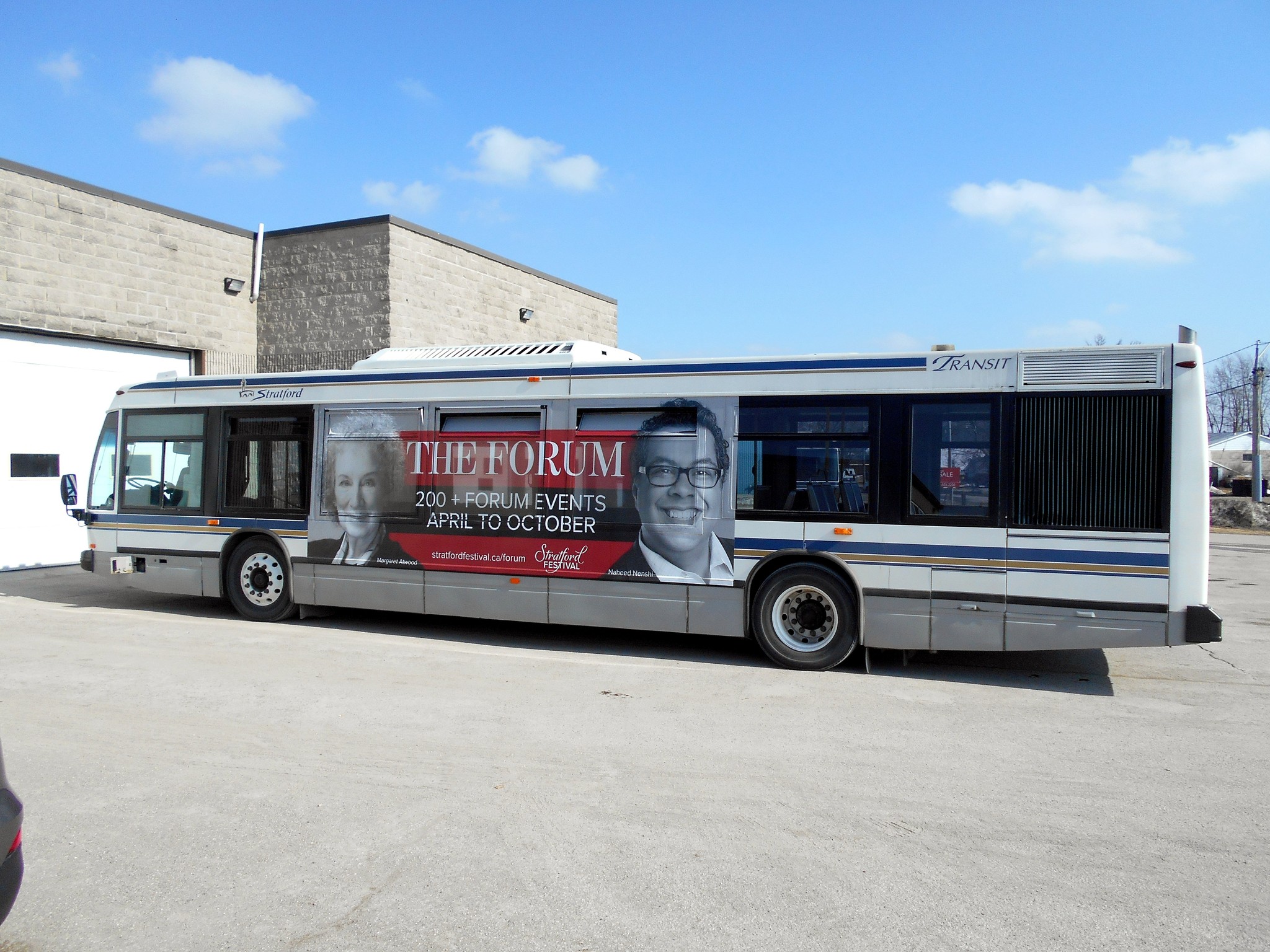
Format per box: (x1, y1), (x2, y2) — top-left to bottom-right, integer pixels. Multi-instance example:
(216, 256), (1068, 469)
(60, 341), (1222, 673)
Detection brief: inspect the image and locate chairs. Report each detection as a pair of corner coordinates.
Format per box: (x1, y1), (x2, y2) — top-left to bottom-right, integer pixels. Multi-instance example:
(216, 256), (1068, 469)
(783, 489), (807, 510)
(840, 480), (866, 512)
(807, 482), (839, 512)
(170, 456), (191, 506)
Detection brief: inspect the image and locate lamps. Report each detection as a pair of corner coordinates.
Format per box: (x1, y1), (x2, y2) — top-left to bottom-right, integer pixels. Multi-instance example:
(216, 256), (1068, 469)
(224, 278), (245, 293)
(520, 308), (533, 320)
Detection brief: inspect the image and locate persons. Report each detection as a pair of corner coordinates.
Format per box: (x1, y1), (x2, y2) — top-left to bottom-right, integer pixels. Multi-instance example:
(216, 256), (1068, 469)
(291, 409), (424, 570)
(598, 397), (734, 586)
